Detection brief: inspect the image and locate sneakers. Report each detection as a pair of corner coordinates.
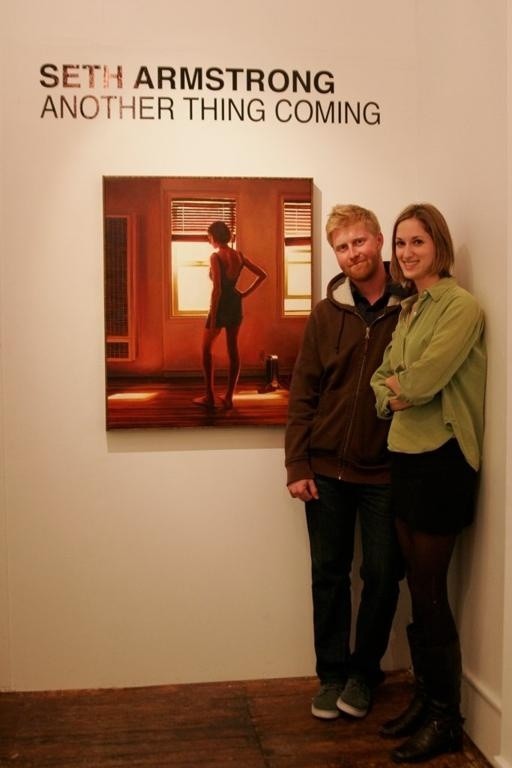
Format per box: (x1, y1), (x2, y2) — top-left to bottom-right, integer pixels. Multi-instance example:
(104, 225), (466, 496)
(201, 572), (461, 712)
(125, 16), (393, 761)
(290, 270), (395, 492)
(311, 679), (347, 719)
(336, 677), (372, 717)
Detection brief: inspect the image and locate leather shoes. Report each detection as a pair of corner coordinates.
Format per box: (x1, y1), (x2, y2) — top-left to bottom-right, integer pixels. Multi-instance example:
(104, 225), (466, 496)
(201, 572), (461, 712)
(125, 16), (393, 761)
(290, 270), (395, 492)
(391, 708), (463, 763)
(377, 696), (430, 736)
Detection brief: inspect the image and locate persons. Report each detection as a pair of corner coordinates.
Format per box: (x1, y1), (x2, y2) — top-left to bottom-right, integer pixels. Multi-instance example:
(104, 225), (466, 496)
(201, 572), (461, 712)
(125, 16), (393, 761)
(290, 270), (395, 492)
(284, 202), (402, 721)
(192, 220), (269, 408)
(369, 201), (490, 764)
